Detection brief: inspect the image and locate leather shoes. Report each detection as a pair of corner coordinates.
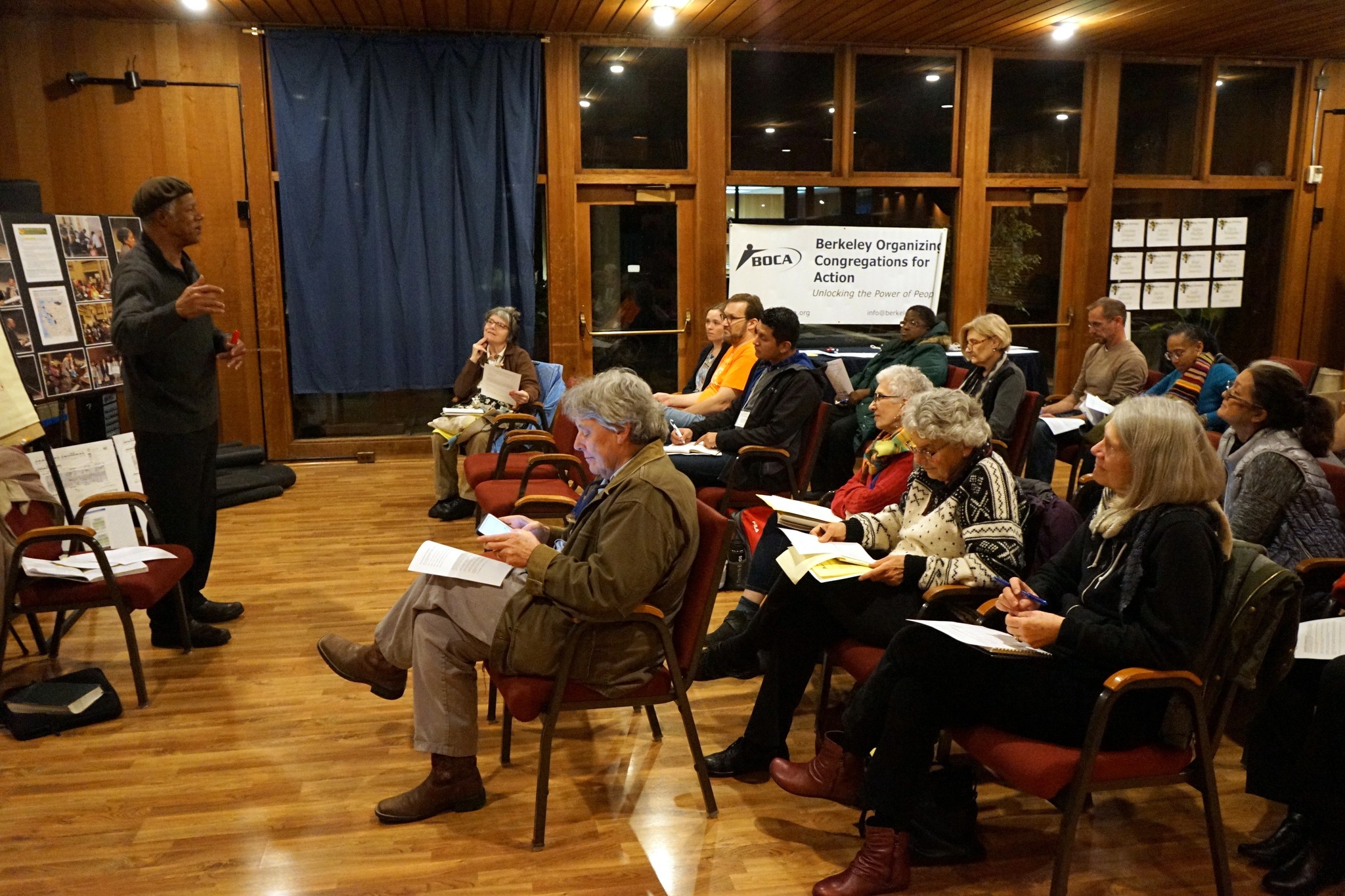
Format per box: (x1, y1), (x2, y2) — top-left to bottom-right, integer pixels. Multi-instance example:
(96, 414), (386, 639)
(704, 736), (788, 779)
(317, 633), (408, 700)
(150, 619), (231, 649)
(189, 597), (245, 623)
(437, 498), (477, 521)
(1238, 810), (1309, 864)
(1262, 846), (1345, 896)
(375, 755), (485, 822)
(692, 639), (763, 682)
(428, 492), (460, 518)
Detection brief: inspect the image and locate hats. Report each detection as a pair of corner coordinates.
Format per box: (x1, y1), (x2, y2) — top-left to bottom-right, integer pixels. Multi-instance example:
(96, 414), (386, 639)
(132, 174), (194, 217)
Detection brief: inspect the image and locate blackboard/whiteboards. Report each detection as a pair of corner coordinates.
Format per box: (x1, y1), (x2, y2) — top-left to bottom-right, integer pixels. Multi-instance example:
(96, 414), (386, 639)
(0, 311), (45, 450)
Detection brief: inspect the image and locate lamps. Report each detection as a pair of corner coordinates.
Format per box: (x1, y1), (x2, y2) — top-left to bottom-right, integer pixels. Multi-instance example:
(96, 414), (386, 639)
(125, 71), (142, 90)
(66, 72), (88, 89)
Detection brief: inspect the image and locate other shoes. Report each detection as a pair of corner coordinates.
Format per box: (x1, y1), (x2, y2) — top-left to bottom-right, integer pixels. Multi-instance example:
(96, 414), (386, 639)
(700, 610), (758, 648)
(802, 490), (823, 501)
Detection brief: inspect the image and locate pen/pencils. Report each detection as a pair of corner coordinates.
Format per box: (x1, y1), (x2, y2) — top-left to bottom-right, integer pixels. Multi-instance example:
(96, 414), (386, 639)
(990, 575), (1047, 606)
(820, 527), (825, 534)
(478, 350), (480, 354)
(670, 419), (686, 444)
(835, 398), (849, 405)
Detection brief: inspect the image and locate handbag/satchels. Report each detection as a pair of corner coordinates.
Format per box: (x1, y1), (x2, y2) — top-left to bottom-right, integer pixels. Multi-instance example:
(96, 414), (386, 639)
(912, 756), (985, 867)
(0, 667), (123, 741)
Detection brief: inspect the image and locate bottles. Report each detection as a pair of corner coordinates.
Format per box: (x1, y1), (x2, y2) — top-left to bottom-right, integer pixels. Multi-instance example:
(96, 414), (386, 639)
(728, 539), (748, 587)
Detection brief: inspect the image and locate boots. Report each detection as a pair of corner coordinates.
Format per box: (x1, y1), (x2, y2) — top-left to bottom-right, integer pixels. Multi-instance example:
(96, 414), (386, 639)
(770, 730), (863, 809)
(812, 814), (911, 896)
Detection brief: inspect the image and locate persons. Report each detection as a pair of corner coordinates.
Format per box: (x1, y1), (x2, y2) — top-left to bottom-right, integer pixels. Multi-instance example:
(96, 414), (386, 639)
(1216, 359), (1345, 688)
(1144, 322), (1238, 435)
(1024, 297), (1149, 484)
(957, 314), (1026, 447)
(1237, 571), (1345, 896)
(0, 217), (137, 395)
(109, 176), (245, 649)
(769, 395), (1232, 896)
(799, 305), (952, 501)
(317, 366), (700, 825)
(652, 293), (764, 428)
(694, 387), (1031, 785)
(668, 307), (837, 493)
(681, 303), (732, 394)
(428, 307), (541, 521)
(704, 364), (934, 647)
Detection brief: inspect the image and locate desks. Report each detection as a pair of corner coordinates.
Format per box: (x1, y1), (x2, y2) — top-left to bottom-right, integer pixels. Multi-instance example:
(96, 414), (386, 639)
(796, 345), (1048, 396)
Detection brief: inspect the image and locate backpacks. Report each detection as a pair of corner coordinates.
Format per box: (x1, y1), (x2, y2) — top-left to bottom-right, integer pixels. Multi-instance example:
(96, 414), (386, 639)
(725, 507), (775, 590)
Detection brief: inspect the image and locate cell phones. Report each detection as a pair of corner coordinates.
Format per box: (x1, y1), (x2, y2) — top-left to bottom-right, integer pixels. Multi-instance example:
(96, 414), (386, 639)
(475, 513), (513, 535)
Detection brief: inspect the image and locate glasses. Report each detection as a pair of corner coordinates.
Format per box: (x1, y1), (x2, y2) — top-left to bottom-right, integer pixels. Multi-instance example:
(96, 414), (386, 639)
(486, 318), (510, 331)
(965, 336), (994, 352)
(719, 313), (749, 323)
(1164, 341), (1196, 360)
(873, 392), (900, 403)
(900, 320), (926, 327)
(906, 437), (951, 459)
(1225, 381), (1267, 409)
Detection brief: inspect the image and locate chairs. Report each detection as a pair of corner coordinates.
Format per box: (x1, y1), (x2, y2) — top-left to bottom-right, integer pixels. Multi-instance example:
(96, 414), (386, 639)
(452, 361), (596, 552)
(0, 447), (193, 708)
(487, 498), (735, 851)
(812, 634), (1231, 896)
(697, 401), (833, 512)
(1267, 356), (1319, 394)
(1009, 387), (1046, 476)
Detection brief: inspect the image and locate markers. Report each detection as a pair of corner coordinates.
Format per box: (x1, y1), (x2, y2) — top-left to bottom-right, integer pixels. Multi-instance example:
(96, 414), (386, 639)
(230, 329), (239, 346)
